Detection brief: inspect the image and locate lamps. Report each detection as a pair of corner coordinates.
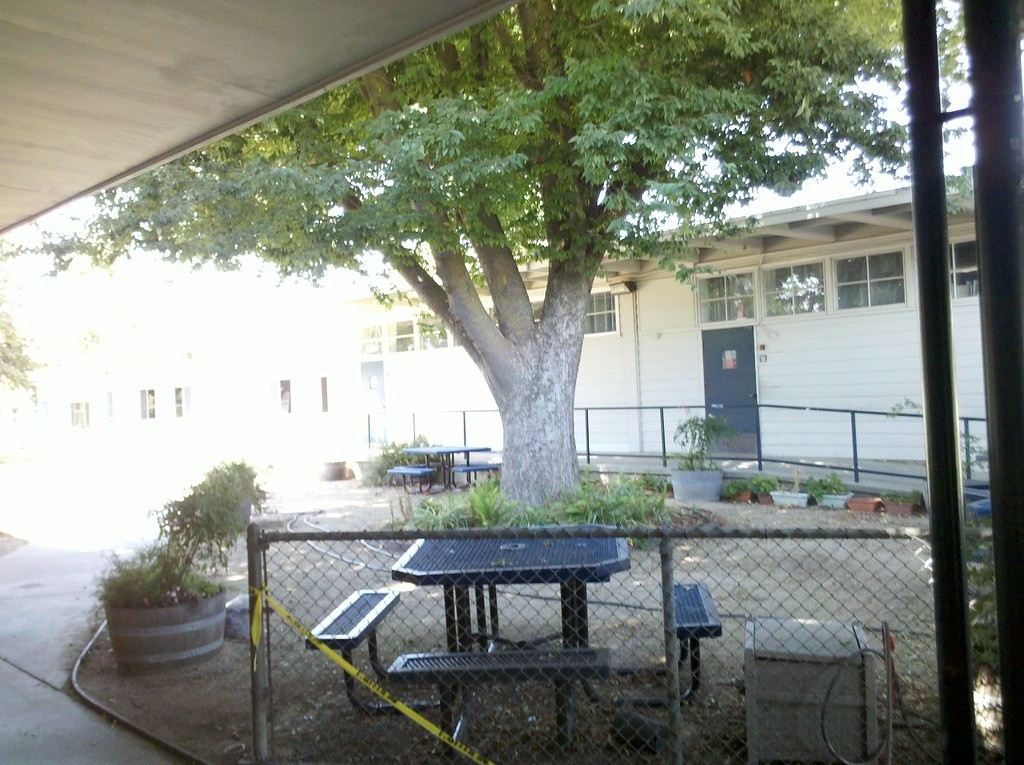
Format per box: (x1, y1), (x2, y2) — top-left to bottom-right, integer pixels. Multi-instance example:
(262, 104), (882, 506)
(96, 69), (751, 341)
(609, 281), (635, 295)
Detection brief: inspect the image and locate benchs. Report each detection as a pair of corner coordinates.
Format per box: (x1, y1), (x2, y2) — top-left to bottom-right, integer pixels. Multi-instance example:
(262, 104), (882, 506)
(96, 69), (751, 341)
(661, 582), (722, 708)
(386, 647), (612, 754)
(489, 574), (610, 638)
(387, 462), (498, 495)
(306, 590), (400, 713)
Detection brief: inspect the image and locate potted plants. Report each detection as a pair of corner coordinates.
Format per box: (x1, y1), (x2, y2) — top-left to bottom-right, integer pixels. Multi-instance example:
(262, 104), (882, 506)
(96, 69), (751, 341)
(769, 470), (811, 507)
(749, 473), (777, 504)
(89, 462), (278, 670)
(880, 490), (924, 517)
(724, 481), (755, 503)
(805, 472), (853, 509)
(671, 415), (741, 502)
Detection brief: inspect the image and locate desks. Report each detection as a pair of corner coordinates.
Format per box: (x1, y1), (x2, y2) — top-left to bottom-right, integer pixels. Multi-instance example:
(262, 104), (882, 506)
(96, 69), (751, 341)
(391, 524), (630, 713)
(402, 447), (491, 494)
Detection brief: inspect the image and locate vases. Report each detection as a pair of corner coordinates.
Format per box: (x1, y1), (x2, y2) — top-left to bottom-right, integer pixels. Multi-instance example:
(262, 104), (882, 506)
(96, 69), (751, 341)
(846, 497), (882, 513)
(324, 461), (347, 481)
